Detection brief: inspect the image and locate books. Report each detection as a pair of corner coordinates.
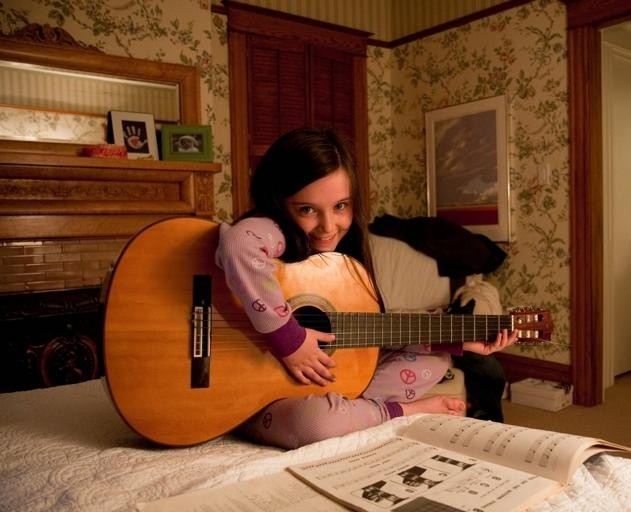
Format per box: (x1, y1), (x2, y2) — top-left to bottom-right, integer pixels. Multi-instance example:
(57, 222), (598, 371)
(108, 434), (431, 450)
(286, 413), (631, 511)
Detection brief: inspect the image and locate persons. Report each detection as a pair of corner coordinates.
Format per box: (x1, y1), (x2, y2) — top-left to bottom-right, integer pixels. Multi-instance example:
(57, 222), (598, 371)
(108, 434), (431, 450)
(215, 127), (519, 450)
(177, 135), (199, 151)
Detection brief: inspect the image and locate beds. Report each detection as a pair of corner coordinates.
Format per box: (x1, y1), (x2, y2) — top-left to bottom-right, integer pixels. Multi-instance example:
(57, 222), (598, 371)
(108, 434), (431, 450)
(0, 376), (631, 512)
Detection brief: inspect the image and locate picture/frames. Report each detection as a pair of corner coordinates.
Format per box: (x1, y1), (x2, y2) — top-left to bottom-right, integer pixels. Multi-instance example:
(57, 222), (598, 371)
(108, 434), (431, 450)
(108, 110), (159, 160)
(162, 123), (214, 162)
(423, 93), (511, 244)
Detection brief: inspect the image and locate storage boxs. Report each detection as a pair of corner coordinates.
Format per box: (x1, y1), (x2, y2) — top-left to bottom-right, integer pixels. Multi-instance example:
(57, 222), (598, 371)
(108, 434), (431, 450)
(510, 377), (574, 412)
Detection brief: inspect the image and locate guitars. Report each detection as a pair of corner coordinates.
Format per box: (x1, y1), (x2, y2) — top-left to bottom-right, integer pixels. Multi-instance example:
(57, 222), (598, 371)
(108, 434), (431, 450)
(104, 218), (553, 448)
